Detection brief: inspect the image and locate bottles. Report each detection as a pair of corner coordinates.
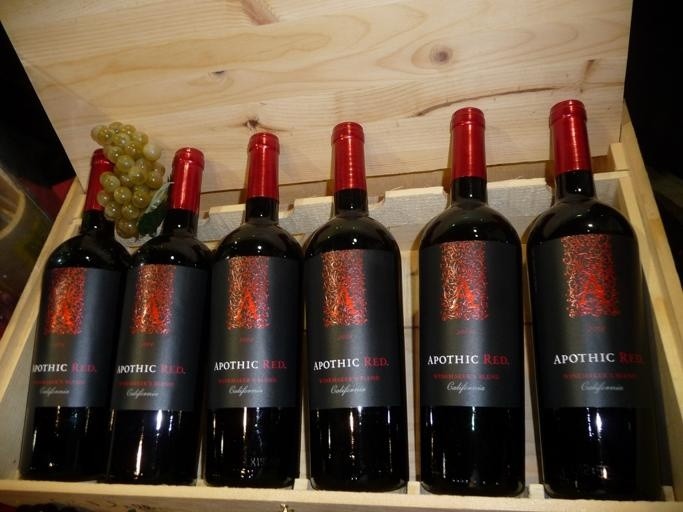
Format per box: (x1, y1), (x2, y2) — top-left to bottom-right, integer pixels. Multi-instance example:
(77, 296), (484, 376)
(526, 98), (666, 502)
(418, 103), (527, 498)
(306, 121), (410, 494)
(17, 147), (131, 484)
(100, 145), (215, 486)
(213, 131), (307, 489)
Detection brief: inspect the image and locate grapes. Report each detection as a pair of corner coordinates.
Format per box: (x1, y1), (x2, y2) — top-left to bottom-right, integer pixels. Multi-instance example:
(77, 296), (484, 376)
(89, 119), (167, 238)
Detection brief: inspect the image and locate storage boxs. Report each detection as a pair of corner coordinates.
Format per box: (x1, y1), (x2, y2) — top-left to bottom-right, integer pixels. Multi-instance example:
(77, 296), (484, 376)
(1, 1), (683, 512)
